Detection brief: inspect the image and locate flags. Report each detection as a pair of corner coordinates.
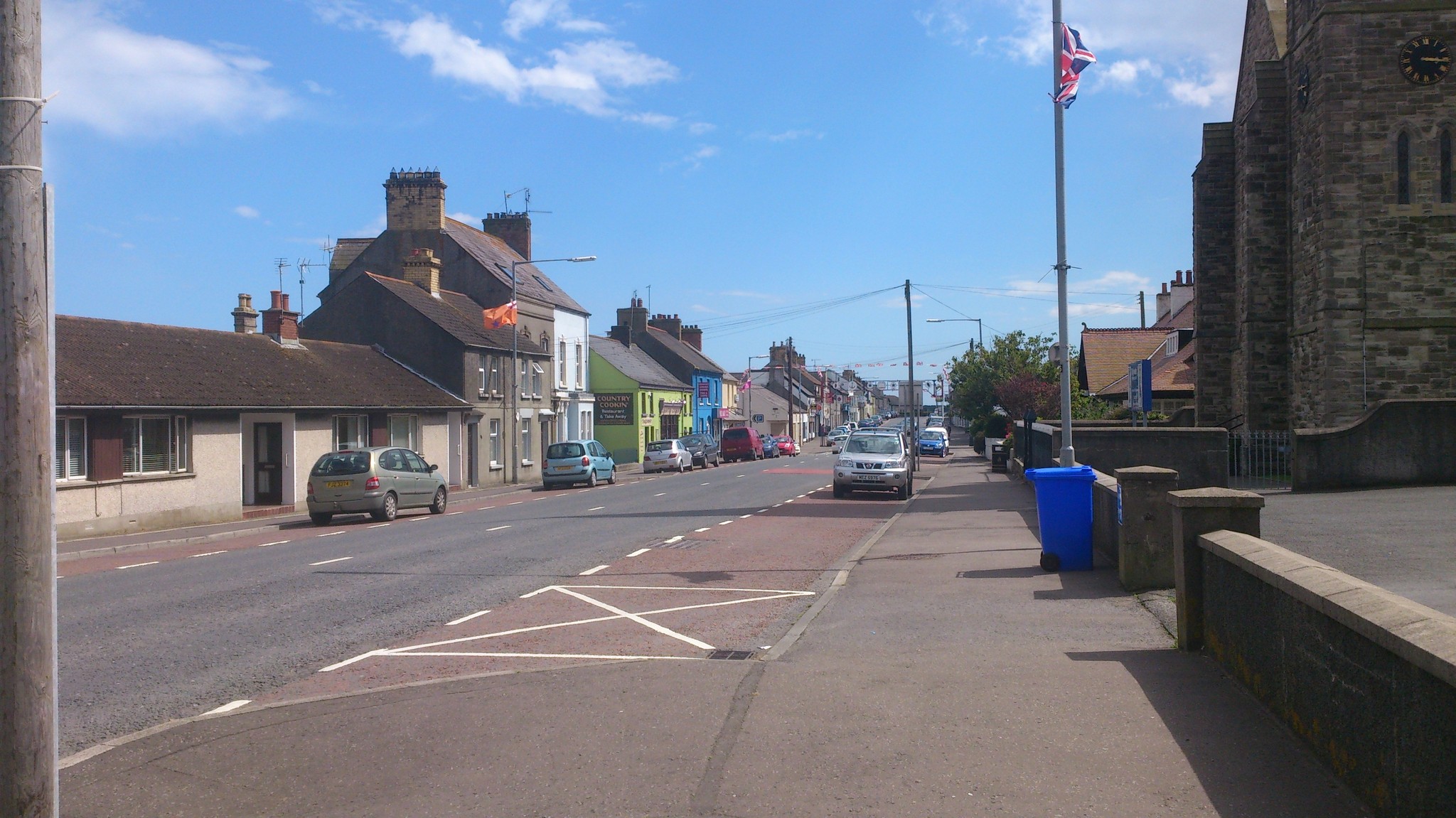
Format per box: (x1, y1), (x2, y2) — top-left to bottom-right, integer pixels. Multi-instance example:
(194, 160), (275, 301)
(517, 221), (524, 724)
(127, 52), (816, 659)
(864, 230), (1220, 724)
(1048, 21), (1097, 109)
(738, 377), (751, 392)
(482, 299), (517, 328)
(818, 361), (937, 376)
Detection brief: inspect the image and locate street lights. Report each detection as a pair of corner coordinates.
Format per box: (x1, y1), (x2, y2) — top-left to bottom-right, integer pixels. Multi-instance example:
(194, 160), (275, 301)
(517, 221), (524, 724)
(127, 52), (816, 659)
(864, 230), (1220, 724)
(799, 365), (830, 447)
(749, 355), (770, 427)
(926, 318), (983, 350)
(512, 255), (598, 485)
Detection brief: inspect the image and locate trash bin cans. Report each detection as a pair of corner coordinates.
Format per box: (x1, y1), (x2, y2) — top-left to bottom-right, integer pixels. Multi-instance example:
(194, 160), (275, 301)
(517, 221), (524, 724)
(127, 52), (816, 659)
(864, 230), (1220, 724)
(1025, 465), (1098, 571)
(992, 445), (1007, 473)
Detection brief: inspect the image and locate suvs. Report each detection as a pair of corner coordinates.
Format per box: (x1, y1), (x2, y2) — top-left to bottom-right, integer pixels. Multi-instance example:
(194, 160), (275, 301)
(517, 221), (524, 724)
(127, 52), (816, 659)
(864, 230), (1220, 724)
(833, 427), (913, 500)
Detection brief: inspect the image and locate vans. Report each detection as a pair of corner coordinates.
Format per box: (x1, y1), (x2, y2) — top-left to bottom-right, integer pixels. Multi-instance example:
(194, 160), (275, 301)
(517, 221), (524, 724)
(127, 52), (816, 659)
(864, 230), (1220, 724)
(722, 426), (765, 463)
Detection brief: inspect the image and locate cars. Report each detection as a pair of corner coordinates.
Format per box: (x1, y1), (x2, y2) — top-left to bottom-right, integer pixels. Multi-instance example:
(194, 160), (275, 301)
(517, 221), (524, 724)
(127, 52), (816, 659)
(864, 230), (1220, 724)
(541, 439), (617, 490)
(679, 433), (720, 469)
(772, 435), (796, 457)
(793, 442), (800, 455)
(825, 408), (951, 458)
(643, 439), (694, 474)
(760, 435), (780, 458)
(306, 447), (448, 524)
